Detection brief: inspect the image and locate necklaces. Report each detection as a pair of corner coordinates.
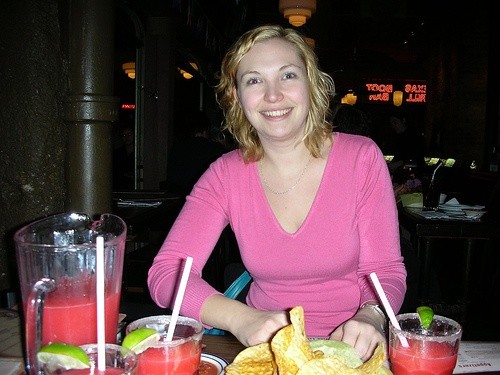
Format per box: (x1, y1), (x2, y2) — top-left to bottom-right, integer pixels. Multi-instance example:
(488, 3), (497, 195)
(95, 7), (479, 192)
(259, 145), (312, 195)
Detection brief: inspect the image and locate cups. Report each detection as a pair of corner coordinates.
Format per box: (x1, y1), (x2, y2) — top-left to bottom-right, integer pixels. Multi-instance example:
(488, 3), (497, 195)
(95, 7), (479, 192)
(44, 343), (138, 375)
(389, 313), (462, 375)
(126, 315), (204, 375)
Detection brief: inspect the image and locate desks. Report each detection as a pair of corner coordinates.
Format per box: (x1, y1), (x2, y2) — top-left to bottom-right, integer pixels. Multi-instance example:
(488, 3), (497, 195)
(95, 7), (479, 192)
(0, 305), (500, 375)
(396, 202), (489, 321)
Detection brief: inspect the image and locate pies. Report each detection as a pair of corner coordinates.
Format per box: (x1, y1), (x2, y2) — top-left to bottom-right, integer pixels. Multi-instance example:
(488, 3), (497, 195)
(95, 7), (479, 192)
(223, 305), (387, 375)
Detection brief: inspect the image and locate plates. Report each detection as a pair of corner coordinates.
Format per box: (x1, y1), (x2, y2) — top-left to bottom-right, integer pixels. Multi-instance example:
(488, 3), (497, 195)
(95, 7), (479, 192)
(440, 205), (485, 214)
(199, 352), (229, 375)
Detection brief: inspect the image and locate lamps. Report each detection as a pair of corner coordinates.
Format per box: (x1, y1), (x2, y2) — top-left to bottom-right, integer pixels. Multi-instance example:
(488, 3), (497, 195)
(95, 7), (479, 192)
(392, 80), (403, 107)
(279, 0), (316, 27)
(340, 89), (357, 106)
(122, 61), (136, 79)
(177, 59), (198, 80)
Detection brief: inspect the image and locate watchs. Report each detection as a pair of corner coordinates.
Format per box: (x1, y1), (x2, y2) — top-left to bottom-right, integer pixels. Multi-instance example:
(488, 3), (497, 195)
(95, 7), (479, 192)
(362, 304), (388, 324)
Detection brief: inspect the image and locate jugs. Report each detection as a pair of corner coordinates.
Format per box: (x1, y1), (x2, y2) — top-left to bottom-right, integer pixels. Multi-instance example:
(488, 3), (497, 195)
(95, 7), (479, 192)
(13, 211), (127, 375)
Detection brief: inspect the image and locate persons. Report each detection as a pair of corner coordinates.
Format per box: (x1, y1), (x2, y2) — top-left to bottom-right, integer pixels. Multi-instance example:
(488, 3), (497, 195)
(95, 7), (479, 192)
(170, 112), (228, 210)
(148, 24), (408, 362)
(384, 110), (426, 182)
(111, 122), (137, 166)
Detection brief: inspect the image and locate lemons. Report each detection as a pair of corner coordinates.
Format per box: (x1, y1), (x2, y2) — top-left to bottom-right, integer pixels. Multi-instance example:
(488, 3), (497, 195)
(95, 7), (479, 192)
(121, 328), (161, 358)
(37, 342), (90, 369)
(417, 306), (433, 329)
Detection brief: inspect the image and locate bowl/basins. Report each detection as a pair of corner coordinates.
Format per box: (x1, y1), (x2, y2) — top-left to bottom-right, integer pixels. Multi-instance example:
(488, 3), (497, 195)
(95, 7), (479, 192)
(464, 209), (486, 218)
